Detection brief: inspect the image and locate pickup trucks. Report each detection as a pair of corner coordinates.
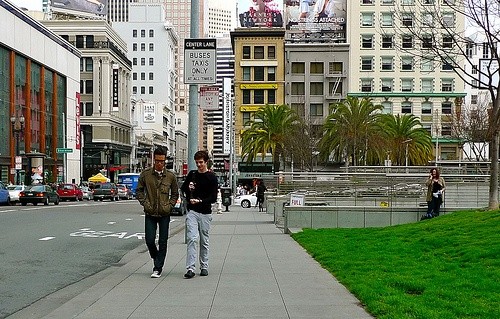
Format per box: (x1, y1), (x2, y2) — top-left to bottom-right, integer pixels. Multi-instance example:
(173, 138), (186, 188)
(92, 182), (119, 201)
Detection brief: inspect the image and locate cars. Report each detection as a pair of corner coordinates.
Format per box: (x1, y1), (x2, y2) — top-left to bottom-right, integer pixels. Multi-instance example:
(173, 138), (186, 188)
(286, 182), (424, 197)
(18, 185), (60, 206)
(233, 189), (274, 208)
(169, 188), (187, 216)
(57, 183), (83, 201)
(7, 184), (28, 206)
(0, 181), (11, 206)
(116, 184), (133, 200)
(81, 187), (94, 201)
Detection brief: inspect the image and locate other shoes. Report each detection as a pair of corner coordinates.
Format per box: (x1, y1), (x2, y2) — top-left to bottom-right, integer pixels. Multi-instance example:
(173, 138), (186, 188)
(184, 270), (195, 278)
(199, 253), (208, 276)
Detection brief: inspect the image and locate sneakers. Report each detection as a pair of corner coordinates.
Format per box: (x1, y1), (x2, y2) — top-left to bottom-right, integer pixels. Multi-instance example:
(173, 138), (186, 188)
(151, 270), (161, 278)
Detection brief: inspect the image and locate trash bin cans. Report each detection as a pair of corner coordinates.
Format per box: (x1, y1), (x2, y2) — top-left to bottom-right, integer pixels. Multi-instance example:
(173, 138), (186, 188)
(221, 188), (231, 212)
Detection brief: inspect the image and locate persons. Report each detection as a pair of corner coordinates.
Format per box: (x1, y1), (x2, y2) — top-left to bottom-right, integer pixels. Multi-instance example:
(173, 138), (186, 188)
(237, 185), (250, 195)
(257, 181), (267, 212)
(136, 147), (179, 278)
(180, 151), (218, 277)
(240, 0), (283, 27)
(425, 168), (446, 217)
(300, 0), (333, 18)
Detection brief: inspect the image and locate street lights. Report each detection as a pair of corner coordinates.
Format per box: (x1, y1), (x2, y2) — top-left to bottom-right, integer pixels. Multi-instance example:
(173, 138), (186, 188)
(219, 93), (233, 188)
(10, 114), (25, 185)
(104, 144), (112, 178)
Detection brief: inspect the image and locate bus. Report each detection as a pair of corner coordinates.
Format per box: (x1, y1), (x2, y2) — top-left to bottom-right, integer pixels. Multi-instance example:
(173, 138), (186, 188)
(117, 172), (141, 198)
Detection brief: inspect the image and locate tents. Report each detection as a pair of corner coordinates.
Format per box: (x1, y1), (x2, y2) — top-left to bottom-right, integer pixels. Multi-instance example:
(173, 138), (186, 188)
(88, 173), (110, 187)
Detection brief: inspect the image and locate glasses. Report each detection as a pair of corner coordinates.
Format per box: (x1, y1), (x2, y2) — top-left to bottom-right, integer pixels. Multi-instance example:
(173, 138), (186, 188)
(155, 159), (165, 164)
